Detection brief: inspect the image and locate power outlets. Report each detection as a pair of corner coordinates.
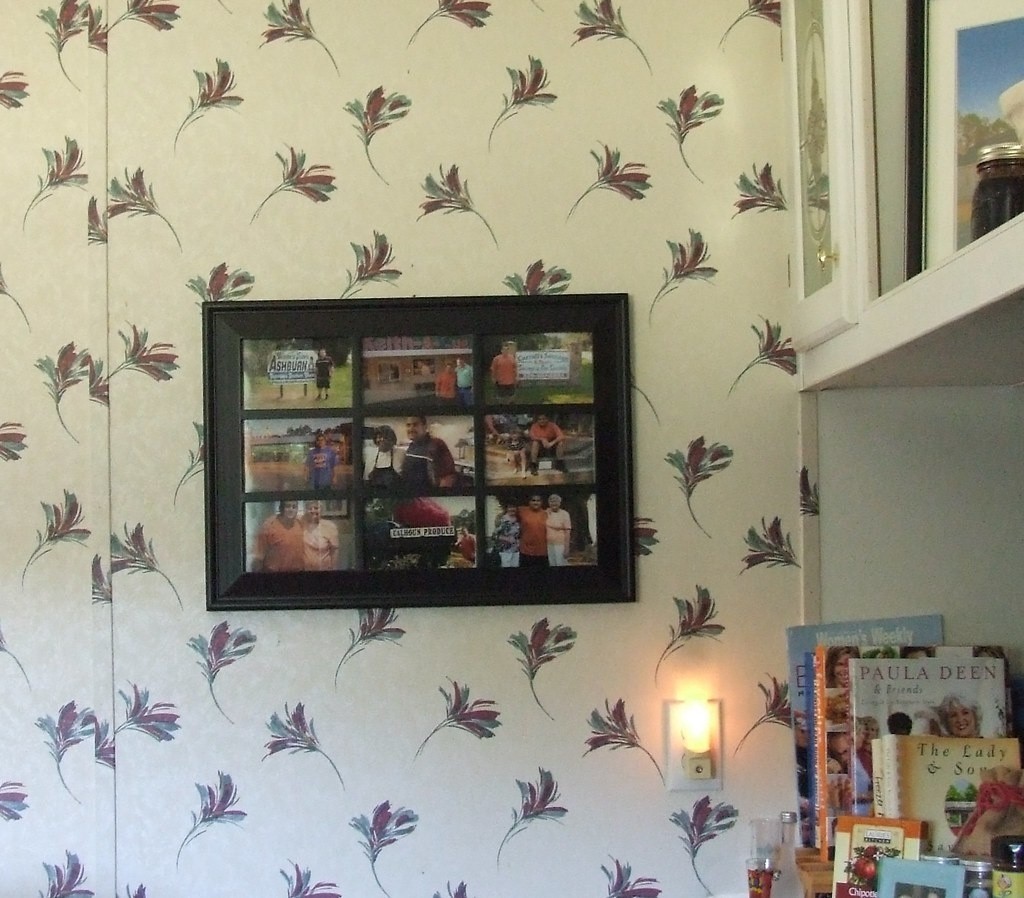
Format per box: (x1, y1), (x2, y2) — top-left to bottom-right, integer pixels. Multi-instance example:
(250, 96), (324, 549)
(664, 699), (724, 793)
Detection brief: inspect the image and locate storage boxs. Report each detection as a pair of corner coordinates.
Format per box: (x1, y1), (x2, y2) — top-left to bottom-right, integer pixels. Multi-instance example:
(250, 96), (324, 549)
(831, 815), (928, 898)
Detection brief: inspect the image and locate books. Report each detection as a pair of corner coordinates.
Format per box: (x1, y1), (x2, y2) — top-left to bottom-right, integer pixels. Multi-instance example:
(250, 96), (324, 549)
(785, 612), (1024, 898)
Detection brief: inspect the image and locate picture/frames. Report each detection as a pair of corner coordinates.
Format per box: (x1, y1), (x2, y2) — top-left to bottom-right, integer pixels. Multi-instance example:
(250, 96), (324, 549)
(200, 294), (637, 612)
(876, 856), (966, 898)
(922, 1), (1024, 271)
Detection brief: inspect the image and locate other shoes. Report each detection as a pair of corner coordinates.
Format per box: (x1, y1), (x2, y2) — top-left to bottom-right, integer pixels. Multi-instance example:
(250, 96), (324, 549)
(513, 468), (518, 473)
(325, 394), (328, 399)
(554, 460), (568, 473)
(529, 462), (539, 475)
(521, 471), (526, 478)
(316, 396), (321, 401)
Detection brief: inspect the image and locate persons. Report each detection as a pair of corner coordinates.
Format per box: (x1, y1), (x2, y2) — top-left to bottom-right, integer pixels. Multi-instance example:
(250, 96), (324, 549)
(830, 732), (850, 773)
(851, 716), (875, 818)
(826, 646), (860, 687)
(871, 649), (887, 659)
(857, 716), (878, 777)
(251, 341), (573, 571)
(827, 755), (845, 775)
(899, 647), (931, 658)
(973, 646), (1012, 688)
(887, 711), (912, 735)
(939, 690), (985, 738)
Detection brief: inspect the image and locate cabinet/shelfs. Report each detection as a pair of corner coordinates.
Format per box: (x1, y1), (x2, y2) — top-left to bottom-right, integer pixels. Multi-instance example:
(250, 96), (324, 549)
(784, 0), (1024, 898)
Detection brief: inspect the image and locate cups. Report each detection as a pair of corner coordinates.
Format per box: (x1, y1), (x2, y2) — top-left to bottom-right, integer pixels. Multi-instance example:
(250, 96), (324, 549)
(744, 858), (773, 898)
(750, 819), (782, 863)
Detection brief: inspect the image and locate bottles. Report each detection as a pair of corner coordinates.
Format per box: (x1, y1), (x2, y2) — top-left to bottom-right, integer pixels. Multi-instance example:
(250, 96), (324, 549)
(770, 811), (805, 898)
(972, 143), (1024, 245)
